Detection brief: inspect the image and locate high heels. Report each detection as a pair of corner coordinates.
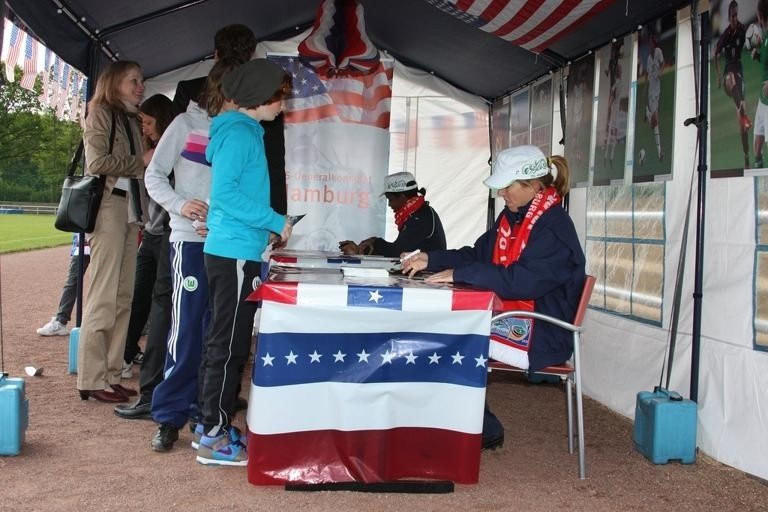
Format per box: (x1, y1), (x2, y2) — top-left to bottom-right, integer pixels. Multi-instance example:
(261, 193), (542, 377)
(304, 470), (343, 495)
(79, 389), (129, 403)
(110, 384), (138, 396)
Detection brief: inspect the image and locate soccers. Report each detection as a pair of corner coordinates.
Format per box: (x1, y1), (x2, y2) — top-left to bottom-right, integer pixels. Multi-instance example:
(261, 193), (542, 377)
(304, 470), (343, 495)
(636, 148), (647, 165)
(744, 24), (765, 48)
(573, 151), (584, 164)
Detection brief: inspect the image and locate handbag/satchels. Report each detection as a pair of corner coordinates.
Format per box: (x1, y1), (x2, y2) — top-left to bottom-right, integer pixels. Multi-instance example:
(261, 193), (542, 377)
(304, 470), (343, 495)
(55, 108), (116, 234)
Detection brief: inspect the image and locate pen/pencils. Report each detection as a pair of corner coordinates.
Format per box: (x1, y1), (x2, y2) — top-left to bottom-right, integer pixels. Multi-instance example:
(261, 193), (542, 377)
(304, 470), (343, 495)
(394, 249), (421, 266)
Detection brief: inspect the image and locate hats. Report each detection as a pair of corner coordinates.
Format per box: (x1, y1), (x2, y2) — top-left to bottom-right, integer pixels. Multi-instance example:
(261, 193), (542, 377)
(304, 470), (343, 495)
(482, 144), (550, 190)
(377, 172), (418, 198)
(222, 57), (286, 107)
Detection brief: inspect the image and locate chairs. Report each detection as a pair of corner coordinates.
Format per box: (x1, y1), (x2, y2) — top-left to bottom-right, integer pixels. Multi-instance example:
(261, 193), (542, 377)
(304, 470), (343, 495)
(486, 274), (598, 485)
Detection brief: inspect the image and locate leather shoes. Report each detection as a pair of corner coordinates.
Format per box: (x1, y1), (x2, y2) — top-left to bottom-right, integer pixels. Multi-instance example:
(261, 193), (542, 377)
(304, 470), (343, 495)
(114, 397), (159, 419)
(231, 396), (248, 411)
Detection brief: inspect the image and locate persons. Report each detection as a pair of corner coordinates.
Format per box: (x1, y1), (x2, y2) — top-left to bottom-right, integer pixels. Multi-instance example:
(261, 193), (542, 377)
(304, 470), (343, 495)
(114, 21), (288, 422)
(567, 74), (589, 167)
(76, 58), (138, 405)
(714, 0), (749, 170)
(142, 53), (239, 453)
(337, 168), (447, 259)
(192, 57), (293, 465)
(113, 92), (180, 380)
(602, 55), (623, 135)
(751, 1), (768, 167)
(643, 36), (667, 165)
(400, 144), (587, 456)
(35, 230), (91, 339)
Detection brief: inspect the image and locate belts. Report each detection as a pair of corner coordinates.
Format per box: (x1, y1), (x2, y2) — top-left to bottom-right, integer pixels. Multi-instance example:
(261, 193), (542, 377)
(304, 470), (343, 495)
(112, 186), (130, 199)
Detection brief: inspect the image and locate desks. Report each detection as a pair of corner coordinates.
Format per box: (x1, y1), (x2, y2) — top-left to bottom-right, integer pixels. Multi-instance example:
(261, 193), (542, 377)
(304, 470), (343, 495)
(246, 248), (497, 495)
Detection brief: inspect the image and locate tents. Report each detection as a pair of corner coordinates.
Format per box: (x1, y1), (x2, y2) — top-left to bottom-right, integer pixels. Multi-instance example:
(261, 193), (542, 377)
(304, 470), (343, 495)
(0, 1), (710, 465)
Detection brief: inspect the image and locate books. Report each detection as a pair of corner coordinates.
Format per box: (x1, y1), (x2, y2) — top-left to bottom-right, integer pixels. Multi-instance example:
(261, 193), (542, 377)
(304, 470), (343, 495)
(268, 263), (406, 287)
(341, 252), (385, 260)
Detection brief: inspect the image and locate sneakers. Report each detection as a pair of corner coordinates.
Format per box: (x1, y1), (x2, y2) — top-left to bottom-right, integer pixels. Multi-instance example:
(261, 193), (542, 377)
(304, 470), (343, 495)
(122, 360), (133, 379)
(37, 317), (69, 335)
(133, 352), (145, 365)
(192, 421), (246, 450)
(196, 434), (249, 466)
(152, 422), (178, 452)
(190, 420), (243, 440)
